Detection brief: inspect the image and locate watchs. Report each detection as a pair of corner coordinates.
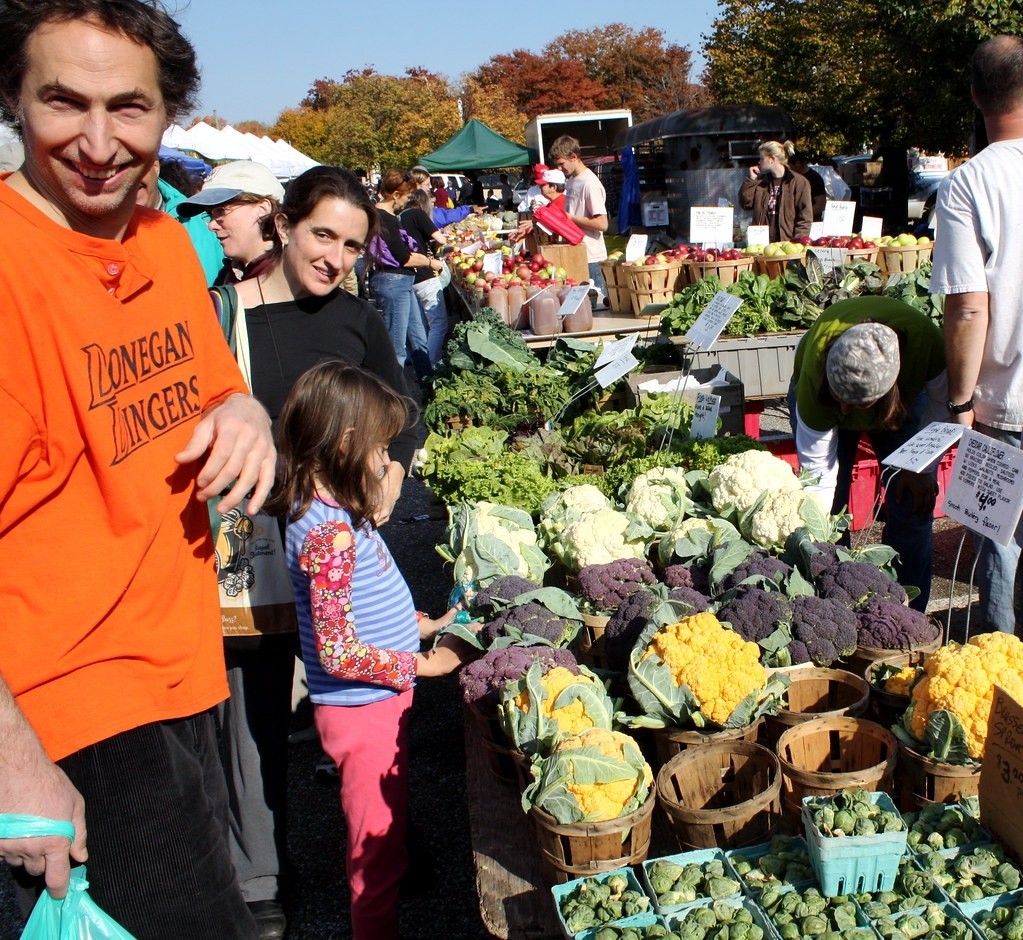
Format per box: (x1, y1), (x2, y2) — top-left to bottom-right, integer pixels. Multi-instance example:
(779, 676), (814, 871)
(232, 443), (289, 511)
(946, 396), (974, 414)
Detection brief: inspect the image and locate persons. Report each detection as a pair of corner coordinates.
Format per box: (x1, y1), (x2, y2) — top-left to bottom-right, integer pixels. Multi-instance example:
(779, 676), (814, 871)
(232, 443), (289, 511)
(928, 32), (1023, 643)
(130, 152), (514, 940)
(263, 358), (486, 940)
(507, 135), (609, 298)
(738, 140), (813, 243)
(787, 149), (826, 222)
(786, 293), (951, 621)
(0, 0), (279, 940)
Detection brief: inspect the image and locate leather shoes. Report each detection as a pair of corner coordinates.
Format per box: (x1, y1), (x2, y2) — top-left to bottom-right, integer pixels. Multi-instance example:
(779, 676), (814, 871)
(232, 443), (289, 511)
(245, 898), (285, 940)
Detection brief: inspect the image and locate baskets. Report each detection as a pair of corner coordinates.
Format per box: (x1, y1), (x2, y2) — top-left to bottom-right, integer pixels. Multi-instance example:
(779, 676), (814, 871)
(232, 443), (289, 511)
(551, 791), (1023, 940)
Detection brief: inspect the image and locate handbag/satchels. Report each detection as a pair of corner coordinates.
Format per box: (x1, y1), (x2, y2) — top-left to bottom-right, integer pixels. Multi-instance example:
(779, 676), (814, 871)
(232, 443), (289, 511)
(446, 197), (455, 209)
(0, 814), (137, 940)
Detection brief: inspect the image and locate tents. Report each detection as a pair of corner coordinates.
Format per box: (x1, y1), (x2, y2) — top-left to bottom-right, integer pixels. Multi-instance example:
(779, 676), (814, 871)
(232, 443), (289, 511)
(419, 118), (534, 171)
(0, 118), (325, 183)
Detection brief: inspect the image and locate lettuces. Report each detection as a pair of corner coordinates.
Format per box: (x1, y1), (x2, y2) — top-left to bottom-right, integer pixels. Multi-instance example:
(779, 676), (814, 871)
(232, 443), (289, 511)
(438, 431), (771, 516)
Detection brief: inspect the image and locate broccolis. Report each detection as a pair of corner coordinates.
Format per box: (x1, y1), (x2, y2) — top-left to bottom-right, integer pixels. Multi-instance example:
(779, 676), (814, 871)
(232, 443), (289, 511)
(438, 446), (939, 668)
(874, 632), (1023, 767)
(493, 610), (789, 826)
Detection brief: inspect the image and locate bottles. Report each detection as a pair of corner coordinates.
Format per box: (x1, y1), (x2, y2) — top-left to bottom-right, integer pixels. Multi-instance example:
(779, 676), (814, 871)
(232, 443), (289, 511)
(488, 278), (593, 335)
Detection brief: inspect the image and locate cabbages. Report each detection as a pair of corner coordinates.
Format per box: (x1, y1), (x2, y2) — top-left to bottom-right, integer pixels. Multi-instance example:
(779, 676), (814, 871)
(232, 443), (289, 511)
(559, 787), (1023, 940)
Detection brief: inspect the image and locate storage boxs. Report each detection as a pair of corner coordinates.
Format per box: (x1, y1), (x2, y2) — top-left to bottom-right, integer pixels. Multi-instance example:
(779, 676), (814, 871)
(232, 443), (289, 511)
(625, 363), (745, 438)
(642, 201), (669, 227)
(743, 400), (958, 533)
(906, 153), (947, 173)
(679, 335), (807, 397)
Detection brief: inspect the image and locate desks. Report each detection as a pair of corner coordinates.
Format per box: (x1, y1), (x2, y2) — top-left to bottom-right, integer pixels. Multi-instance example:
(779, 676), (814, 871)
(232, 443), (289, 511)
(482, 229), (525, 252)
(448, 280), (664, 350)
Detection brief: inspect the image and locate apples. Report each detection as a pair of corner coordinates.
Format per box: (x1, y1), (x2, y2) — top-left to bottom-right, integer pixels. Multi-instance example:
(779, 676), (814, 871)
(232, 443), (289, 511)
(445, 244), (576, 292)
(606, 233), (930, 284)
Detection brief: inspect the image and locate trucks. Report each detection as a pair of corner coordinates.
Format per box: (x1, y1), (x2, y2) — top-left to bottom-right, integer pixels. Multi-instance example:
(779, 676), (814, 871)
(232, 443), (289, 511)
(524, 108), (634, 171)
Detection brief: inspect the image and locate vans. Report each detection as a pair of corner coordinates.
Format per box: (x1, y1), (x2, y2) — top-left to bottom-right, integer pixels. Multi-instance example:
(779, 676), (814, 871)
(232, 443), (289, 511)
(476, 174), (519, 188)
(427, 172), (473, 192)
(581, 102), (800, 237)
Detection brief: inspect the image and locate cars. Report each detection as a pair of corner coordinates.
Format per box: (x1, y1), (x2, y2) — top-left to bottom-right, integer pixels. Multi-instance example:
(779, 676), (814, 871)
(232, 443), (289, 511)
(510, 179), (530, 210)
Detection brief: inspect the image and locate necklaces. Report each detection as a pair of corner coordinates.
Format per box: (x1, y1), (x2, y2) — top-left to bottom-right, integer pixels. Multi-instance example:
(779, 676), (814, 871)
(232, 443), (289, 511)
(257, 270), (286, 379)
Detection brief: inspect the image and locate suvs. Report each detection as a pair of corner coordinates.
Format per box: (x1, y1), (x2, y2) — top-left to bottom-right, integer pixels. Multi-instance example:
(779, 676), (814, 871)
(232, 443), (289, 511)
(830, 154), (951, 240)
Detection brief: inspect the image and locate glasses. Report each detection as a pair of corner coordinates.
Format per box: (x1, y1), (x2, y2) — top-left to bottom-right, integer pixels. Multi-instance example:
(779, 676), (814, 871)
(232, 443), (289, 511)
(415, 181), (430, 189)
(202, 200), (258, 223)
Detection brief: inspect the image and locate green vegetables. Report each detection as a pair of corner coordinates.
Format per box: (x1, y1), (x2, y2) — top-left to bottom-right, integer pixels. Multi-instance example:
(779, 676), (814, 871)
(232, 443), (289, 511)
(417, 310), (723, 480)
(656, 250), (942, 337)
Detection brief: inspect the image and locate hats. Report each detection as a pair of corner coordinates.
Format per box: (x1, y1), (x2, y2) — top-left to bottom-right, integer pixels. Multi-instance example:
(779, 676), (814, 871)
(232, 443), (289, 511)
(534, 169), (567, 185)
(176, 161), (287, 217)
(533, 164), (550, 178)
(826, 321), (899, 402)
